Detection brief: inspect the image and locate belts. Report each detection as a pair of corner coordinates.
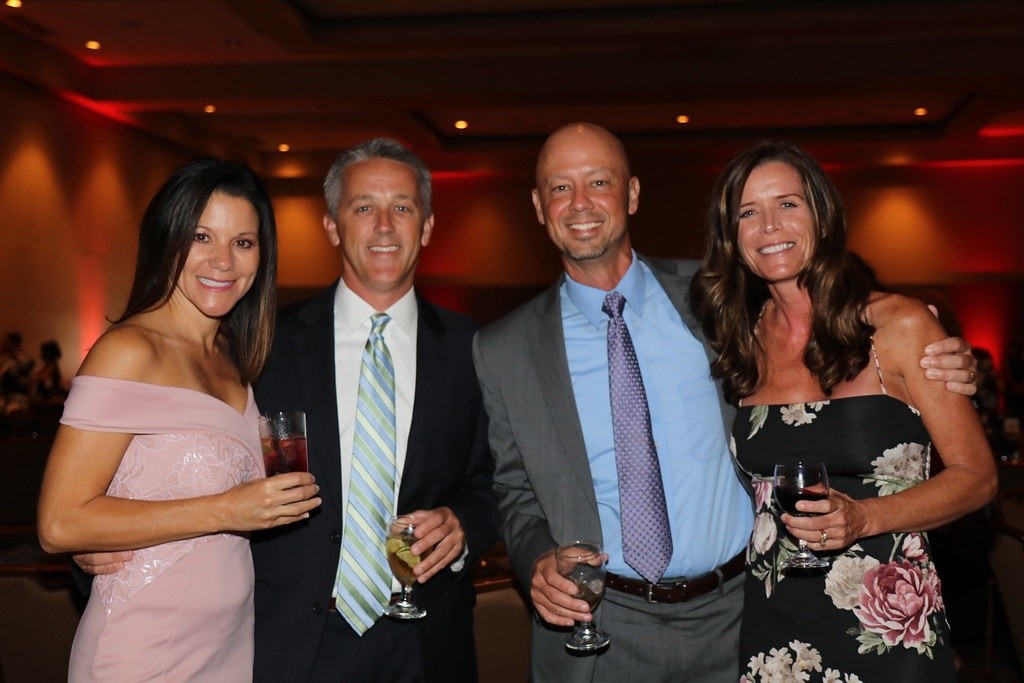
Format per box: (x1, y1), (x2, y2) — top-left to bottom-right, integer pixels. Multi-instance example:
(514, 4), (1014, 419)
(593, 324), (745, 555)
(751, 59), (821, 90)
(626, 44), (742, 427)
(606, 547), (747, 603)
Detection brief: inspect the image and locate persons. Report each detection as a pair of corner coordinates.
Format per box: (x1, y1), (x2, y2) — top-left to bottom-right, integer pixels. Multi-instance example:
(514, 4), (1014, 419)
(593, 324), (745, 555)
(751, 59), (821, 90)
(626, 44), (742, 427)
(0, 329), (36, 398)
(38, 151), (322, 683)
(34, 340), (65, 411)
(72, 139), (498, 683)
(472, 118), (982, 683)
(691, 139), (999, 683)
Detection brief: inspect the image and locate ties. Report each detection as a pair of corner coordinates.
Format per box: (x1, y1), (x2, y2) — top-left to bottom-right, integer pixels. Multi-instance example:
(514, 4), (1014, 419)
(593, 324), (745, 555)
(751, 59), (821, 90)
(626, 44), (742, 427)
(602, 291), (673, 585)
(334, 312), (397, 636)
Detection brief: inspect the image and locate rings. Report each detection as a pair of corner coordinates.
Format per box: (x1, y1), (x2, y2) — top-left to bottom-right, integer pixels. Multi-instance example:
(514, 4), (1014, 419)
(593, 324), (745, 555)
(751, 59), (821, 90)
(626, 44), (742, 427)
(969, 369), (975, 381)
(818, 528), (827, 546)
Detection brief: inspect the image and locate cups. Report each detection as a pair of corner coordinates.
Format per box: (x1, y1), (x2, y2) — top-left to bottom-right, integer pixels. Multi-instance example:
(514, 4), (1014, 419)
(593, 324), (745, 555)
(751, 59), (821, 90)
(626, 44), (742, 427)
(258, 412), (308, 477)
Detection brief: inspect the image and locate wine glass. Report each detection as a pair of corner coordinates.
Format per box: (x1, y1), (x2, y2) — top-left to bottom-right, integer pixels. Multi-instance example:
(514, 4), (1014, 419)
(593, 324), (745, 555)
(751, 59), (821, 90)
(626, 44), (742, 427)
(382, 515), (432, 618)
(555, 540), (610, 651)
(773, 459), (830, 567)
